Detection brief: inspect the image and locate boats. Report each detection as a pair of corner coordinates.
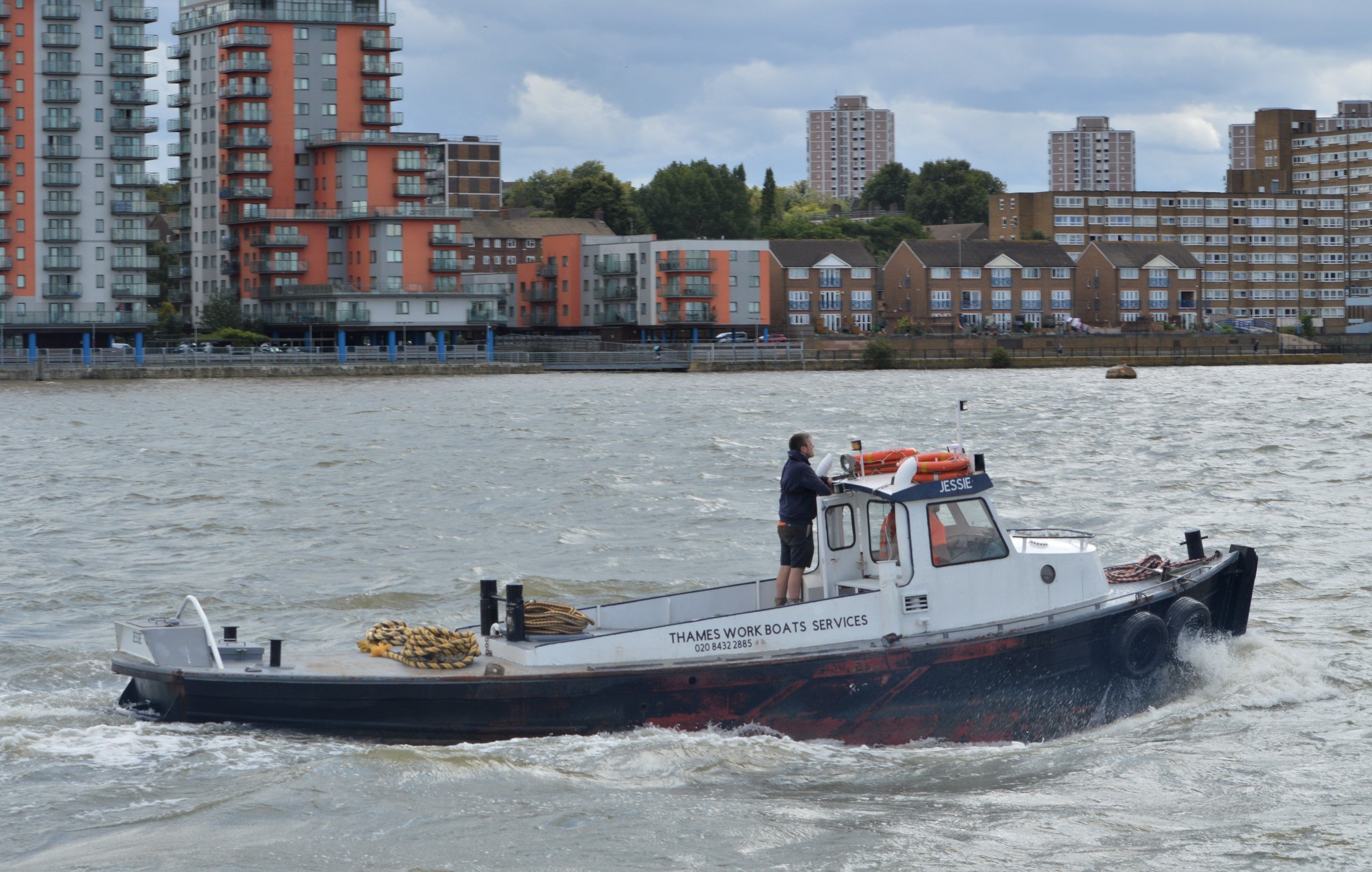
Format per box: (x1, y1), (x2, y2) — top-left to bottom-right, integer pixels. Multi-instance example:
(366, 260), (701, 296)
(111, 440), (1258, 752)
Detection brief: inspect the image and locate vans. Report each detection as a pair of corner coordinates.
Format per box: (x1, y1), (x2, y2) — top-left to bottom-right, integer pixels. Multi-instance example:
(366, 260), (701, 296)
(112, 343), (132, 352)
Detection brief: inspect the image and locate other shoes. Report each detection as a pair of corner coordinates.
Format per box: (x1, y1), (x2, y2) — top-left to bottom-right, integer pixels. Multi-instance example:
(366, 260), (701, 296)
(774, 598), (787, 606)
(788, 599), (802, 604)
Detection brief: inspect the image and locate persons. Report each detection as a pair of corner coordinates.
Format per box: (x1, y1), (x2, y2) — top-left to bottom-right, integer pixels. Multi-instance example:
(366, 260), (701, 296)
(1253, 339), (1259, 354)
(774, 433), (835, 607)
(1058, 342), (1065, 357)
(656, 346), (663, 359)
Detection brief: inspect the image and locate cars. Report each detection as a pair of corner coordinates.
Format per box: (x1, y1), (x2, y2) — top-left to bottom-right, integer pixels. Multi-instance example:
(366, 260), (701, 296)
(749, 334), (788, 348)
(711, 332), (748, 343)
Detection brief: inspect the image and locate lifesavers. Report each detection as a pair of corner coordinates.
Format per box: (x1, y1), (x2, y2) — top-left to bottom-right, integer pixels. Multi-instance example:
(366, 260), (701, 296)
(897, 452), (973, 482)
(1110, 610), (1170, 680)
(850, 447), (918, 476)
(1167, 595), (1212, 667)
